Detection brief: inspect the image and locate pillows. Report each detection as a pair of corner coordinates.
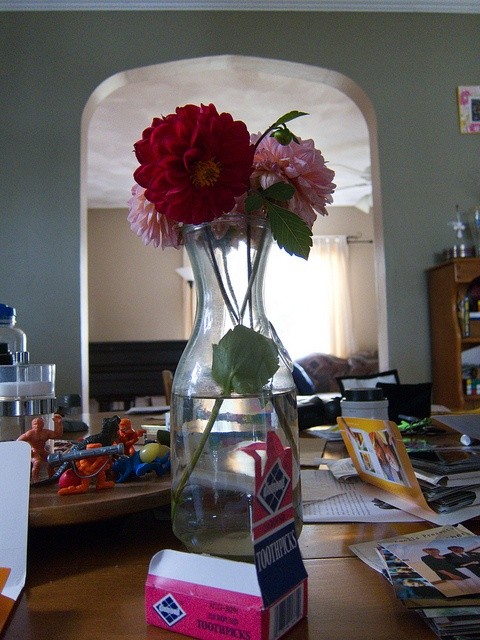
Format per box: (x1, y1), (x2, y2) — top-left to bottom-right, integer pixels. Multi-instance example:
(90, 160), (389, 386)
(293, 362), (314, 391)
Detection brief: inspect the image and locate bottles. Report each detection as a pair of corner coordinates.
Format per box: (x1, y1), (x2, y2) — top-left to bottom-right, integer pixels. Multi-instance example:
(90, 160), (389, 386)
(0, 303), (27, 367)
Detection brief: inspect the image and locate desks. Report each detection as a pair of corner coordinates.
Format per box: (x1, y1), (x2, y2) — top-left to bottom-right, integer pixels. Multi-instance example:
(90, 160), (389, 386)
(0, 413), (479, 639)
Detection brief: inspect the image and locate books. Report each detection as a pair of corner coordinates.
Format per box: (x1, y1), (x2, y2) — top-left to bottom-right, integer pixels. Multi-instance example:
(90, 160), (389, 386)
(462, 365), (480, 396)
(456, 297), (470, 338)
(348, 525), (480, 640)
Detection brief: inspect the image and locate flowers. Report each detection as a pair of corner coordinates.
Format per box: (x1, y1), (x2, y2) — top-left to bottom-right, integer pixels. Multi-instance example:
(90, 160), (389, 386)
(125, 106), (336, 265)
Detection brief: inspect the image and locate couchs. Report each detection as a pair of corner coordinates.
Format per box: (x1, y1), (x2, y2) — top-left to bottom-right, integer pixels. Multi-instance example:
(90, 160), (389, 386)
(294, 350), (379, 431)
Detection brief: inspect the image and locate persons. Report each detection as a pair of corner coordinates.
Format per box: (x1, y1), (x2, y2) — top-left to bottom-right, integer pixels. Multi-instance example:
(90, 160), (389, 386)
(420, 546), (477, 581)
(369, 432), (400, 472)
(16, 415), (63, 485)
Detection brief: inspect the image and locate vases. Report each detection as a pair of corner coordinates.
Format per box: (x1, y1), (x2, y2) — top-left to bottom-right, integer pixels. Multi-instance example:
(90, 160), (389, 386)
(169, 221), (304, 553)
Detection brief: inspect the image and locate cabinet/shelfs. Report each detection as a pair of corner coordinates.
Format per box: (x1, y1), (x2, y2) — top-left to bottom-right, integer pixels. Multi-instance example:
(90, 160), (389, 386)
(424, 259), (480, 412)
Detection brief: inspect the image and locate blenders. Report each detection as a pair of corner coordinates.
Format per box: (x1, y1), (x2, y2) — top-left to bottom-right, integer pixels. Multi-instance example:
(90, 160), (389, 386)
(0, 350), (59, 485)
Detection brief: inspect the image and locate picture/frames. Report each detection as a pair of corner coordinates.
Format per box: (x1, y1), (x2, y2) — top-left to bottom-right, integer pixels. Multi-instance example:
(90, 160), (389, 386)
(455, 83), (479, 134)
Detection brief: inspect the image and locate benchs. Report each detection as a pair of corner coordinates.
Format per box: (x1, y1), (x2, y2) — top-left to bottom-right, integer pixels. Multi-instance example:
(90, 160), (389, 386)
(88, 340), (188, 410)
